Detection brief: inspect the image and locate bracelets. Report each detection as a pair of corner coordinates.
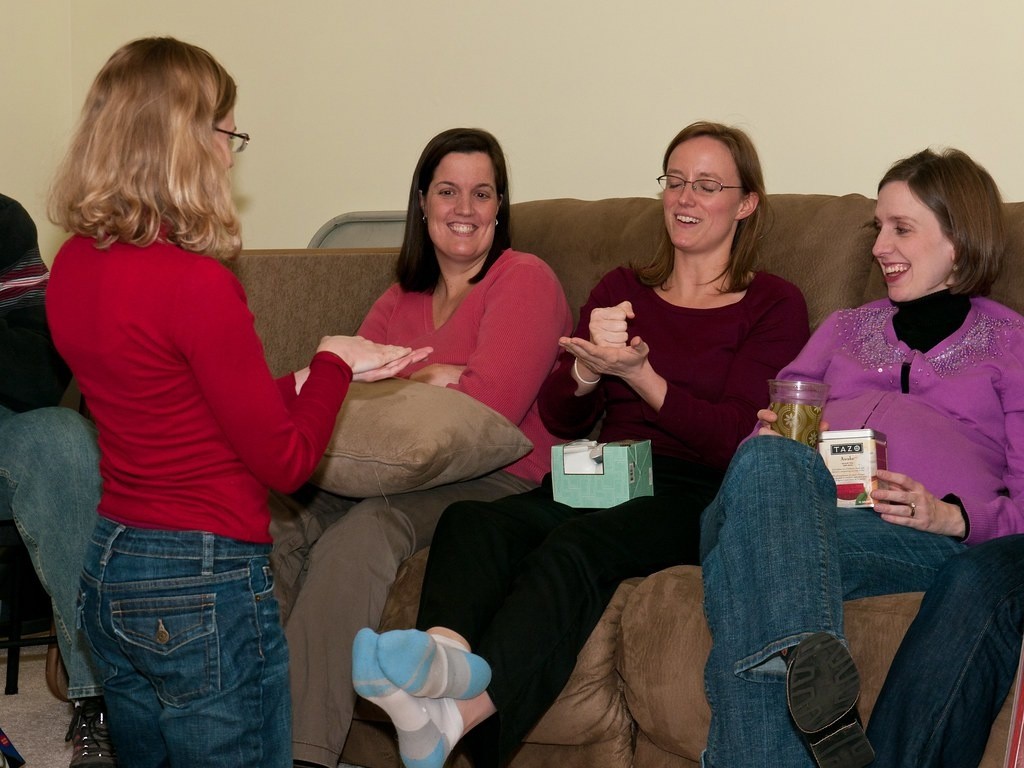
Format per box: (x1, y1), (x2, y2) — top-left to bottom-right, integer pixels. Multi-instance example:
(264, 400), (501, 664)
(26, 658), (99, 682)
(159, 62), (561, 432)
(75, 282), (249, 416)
(574, 356), (601, 385)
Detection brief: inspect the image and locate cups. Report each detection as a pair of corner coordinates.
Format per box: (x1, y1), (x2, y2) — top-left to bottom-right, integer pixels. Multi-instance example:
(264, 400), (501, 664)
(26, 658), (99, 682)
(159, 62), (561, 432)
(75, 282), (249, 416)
(768, 380), (831, 450)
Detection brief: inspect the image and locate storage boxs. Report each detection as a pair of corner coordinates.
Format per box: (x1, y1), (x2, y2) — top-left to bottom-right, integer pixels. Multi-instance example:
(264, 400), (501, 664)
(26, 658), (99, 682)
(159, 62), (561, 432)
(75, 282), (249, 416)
(551, 438), (654, 509)
(817, 428), (890, 508)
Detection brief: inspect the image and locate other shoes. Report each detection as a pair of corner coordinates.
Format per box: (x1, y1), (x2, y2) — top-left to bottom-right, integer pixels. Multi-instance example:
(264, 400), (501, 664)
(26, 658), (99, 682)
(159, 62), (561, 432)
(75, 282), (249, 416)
(785, 631), (877, 768)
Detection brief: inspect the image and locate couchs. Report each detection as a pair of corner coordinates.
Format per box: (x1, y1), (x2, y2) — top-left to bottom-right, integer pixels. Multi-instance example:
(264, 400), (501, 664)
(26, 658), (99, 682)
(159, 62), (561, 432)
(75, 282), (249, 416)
(232, 193), (1024, 768)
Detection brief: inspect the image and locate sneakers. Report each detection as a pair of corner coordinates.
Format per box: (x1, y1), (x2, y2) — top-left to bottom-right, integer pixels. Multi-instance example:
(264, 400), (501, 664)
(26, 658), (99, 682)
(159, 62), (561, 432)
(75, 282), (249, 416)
(65, 695), (121, 768)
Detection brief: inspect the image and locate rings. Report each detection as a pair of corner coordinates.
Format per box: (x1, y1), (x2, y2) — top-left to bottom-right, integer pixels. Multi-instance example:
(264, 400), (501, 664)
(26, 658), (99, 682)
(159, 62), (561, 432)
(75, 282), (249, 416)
(910, 502), (916, 517)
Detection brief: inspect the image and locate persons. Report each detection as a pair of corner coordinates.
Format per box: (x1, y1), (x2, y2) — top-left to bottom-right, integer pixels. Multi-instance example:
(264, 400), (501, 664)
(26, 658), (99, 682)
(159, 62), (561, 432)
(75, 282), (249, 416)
(350, 121), (812, 768)
(43, 35), (435, 768)
(267, 127), (577, 767)
(698, 146), (1024, 768)
(0, 190), (117, 768)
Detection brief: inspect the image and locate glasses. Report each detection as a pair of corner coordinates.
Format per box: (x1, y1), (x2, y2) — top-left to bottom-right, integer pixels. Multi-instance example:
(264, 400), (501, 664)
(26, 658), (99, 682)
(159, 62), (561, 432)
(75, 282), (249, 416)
(656, 174), (747, 197)
(214, 127), (250, 154)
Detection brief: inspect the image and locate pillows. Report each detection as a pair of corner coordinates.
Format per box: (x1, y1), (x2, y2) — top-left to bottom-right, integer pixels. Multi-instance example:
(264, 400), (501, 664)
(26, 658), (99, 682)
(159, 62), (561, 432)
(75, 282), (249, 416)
(308, 379), (534, 498)
